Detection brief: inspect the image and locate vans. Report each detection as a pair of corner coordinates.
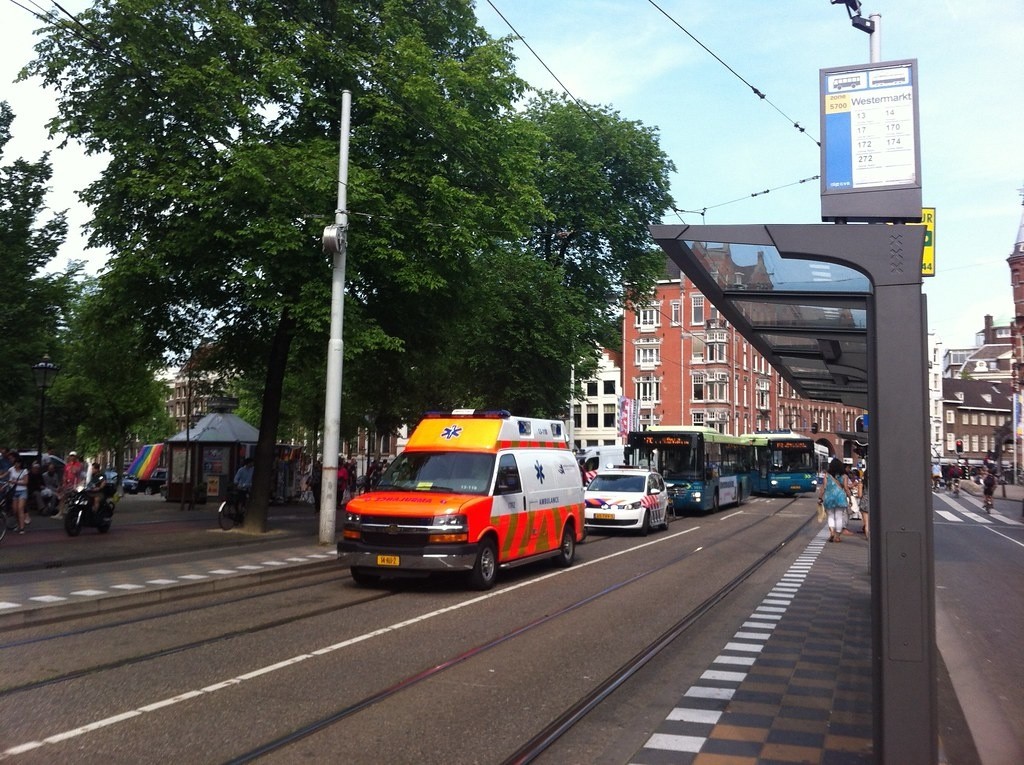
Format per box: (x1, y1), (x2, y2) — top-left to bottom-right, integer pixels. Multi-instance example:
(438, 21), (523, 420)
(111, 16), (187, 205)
(575, 444), (658, 488)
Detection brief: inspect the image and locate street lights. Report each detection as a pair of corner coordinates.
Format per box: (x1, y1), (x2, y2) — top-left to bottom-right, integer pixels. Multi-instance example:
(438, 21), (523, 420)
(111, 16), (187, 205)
(33, 349), (63, 480)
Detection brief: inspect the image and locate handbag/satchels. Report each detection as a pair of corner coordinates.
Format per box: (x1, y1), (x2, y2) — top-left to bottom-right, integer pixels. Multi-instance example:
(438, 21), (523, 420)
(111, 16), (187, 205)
(846, 506), (855, 515)
(341, 489), (352, 505)
(306, 476), (318, 486)
(5, 469), (24, 505)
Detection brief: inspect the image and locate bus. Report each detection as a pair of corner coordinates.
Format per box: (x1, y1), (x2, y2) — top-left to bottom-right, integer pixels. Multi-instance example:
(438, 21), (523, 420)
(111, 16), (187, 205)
(738, 428), (817, 496)
(812, 443), (830, 484)
(623, 426), (753, 515)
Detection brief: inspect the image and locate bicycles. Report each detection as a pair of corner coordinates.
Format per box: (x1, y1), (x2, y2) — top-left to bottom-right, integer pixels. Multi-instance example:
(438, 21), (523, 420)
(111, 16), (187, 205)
(0, 479), (19, 540)
(216, 488), (251, 531)
(982, 493), (994, 516)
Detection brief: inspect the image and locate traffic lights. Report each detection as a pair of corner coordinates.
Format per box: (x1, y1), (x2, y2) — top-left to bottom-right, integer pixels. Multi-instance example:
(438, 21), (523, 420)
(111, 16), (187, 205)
(954, 439), (964, 453)
(855, 415), (869, 447)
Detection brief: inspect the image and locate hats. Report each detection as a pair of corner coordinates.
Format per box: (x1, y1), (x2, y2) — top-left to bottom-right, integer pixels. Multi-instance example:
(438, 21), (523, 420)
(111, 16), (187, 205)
(817, 503), (826, 523)
(350, 458), (357, 465)
(69, 451), (77, 456)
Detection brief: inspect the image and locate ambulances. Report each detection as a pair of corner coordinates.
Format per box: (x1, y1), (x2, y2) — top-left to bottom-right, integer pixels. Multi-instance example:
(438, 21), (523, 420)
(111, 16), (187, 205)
(334, 408), (587, 588)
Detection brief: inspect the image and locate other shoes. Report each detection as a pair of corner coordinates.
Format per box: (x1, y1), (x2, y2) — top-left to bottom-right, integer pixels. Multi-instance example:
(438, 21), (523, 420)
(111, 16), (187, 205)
(24, 519), (31, 524)
(18, 529), (25, 535)
(13, 526), (20, 532)
(50, 515), (63, 520)
(828, 535), (835, 542)
(835, 538), (841, 542)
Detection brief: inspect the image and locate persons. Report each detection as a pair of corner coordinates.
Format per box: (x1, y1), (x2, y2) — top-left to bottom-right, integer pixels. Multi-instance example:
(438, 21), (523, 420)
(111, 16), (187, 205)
(931, 462), (943, 488)
(0, 445), (107, 535)
(982, 468), (998, 509)
(851, 448), (867, 506)
(233, 458), (255, 526)
(307, 457), (411, 516)
(840, 463), (858, 535)
(816, 458), (853, 542)
(948, 463), (963, 494)
(849, 469), (864, 520)
(858, 469), (870, 540)
(960, 459), (1022, 486)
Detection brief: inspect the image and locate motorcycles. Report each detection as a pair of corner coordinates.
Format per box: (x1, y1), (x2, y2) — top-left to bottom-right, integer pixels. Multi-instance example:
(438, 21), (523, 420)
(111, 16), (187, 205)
(62, 475), (117, 536)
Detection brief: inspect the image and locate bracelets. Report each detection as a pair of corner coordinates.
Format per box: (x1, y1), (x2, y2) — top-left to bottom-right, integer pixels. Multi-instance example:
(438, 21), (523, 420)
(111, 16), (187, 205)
(818, 497), (822, 500)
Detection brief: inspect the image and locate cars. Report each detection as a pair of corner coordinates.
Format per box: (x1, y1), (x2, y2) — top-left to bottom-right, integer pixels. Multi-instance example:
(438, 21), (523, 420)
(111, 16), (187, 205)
(104, 469), (119, 490)
(584, 464), (670, 537)
(121, 477), (139, 493)
